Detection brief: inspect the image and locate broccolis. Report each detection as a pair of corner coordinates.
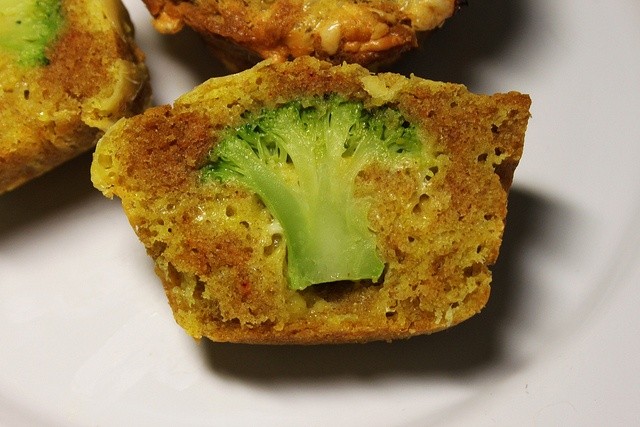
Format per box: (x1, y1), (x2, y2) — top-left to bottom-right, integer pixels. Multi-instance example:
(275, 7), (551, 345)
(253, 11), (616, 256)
(0, 0), (64, 67)
(201, 97), (421, 290)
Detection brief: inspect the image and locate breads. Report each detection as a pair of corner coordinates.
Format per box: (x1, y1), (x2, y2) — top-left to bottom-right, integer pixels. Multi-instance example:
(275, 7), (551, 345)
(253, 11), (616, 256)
(1, 0), (149, 196)
(141, 0), (459, 73)
(91, 57), (532, 344)
